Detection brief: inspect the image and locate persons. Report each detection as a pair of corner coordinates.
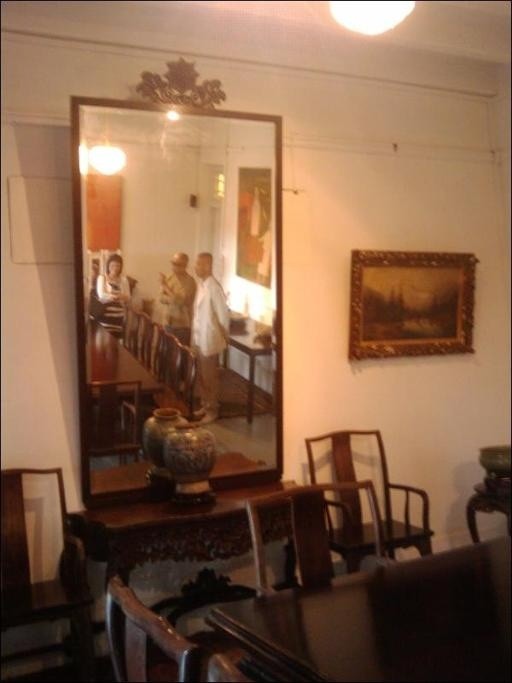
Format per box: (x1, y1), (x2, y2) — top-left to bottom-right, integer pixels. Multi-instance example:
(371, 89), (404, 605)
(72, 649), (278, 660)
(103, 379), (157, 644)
(95, 252), (132, 341)
(156, 251), (199, 393)
(190, 251), (232, 425)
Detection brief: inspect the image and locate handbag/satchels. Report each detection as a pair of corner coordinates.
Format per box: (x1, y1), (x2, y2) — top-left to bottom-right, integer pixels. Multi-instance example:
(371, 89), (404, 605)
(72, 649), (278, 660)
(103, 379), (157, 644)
(89, 272), (110, 322)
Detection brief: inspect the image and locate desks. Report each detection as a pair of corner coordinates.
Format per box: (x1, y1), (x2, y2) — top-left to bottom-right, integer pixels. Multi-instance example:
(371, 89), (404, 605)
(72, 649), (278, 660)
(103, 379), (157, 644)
(228, 310), (276, 425)
(467, 482), (511, 543)
(73, 480), (304, 603)
(204, 538), (510, 680)
(85, 313), (159, 400)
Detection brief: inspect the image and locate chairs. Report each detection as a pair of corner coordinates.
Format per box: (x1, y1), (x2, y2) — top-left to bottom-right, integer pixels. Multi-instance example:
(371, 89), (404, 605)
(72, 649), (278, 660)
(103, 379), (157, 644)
(1, 464), (98, 683)
(88, 382), (143, 466)
(245, 429), (435, 595)
(121, 301), (196, 418)
(101, 574), (199, 682)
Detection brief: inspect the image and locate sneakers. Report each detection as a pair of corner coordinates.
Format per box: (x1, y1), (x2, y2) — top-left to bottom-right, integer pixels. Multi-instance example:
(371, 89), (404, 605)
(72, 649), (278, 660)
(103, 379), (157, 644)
(191, 406), (220, 424)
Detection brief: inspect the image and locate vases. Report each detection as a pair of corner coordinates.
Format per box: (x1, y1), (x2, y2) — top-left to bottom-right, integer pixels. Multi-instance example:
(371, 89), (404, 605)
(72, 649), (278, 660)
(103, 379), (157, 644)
(141, 407), (187, 485)
(162, 423), (220, 507)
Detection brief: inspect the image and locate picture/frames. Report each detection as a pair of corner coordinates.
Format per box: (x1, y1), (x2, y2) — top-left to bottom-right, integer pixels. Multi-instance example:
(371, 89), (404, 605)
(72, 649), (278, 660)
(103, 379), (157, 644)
(346, 249), (476, 363)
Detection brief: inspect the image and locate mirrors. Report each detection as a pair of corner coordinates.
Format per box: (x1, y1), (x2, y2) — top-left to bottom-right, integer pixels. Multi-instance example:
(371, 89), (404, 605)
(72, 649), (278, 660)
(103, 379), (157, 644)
(71, 57), (285, 510)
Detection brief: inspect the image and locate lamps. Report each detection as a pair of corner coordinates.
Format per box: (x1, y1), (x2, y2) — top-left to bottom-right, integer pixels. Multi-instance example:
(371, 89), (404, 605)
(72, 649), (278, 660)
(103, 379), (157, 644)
(91, 107), (126, 175)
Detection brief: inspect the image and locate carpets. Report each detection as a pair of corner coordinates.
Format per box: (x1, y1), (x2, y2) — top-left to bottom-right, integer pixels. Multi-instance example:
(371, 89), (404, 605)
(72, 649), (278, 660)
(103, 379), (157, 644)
(195, 364), (274, 422)
